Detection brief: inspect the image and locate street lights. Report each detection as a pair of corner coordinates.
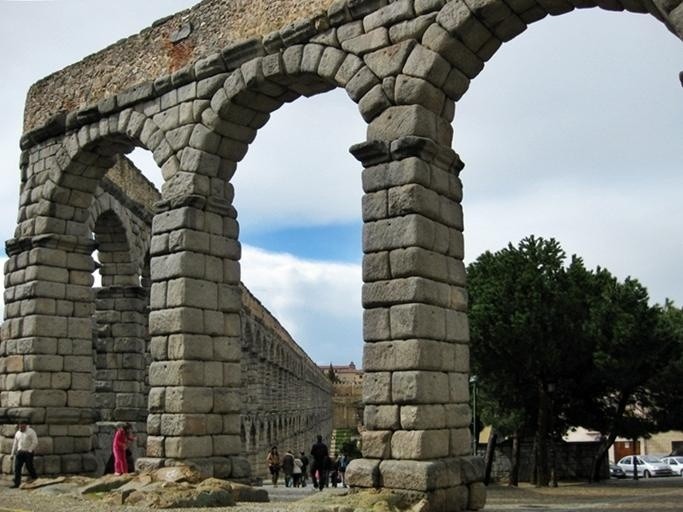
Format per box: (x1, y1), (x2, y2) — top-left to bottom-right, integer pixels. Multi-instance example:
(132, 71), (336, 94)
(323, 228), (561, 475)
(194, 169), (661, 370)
(468, 374), (480, 456)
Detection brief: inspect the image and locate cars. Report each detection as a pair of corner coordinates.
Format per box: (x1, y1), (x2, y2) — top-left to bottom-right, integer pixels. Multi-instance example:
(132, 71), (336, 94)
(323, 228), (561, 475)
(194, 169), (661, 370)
(660, 456), (683, 475)
(617, 454), (672, 477)
(609, 460), (625, 476)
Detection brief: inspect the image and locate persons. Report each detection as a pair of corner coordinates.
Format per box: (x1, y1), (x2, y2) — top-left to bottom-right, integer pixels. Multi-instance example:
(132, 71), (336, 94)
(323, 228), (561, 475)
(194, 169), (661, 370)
(266, 435), (363, 492)
(112, 422), (138, 476)
(9, 420), (39, 489)
